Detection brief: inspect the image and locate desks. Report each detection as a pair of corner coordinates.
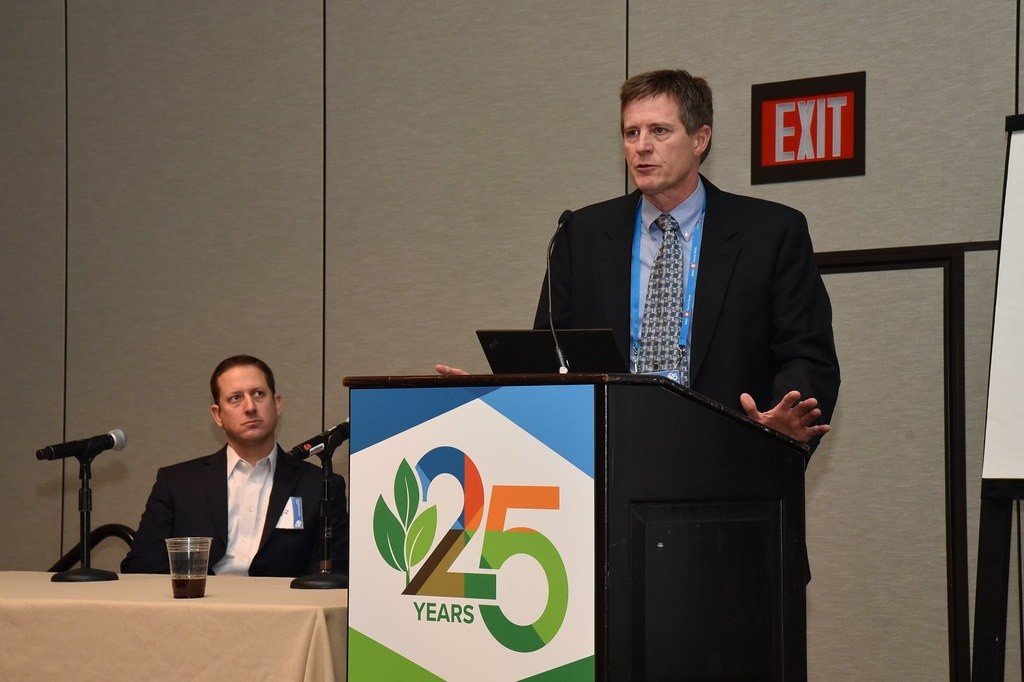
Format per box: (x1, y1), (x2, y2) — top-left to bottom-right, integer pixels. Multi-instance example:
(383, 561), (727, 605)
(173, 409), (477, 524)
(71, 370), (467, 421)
(0, 570), (347, 681)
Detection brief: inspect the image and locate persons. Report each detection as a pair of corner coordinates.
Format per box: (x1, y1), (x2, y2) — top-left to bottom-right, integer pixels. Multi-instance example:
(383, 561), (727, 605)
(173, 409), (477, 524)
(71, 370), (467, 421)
(120, 355), (349, 578)
(434, 70), (841, 472)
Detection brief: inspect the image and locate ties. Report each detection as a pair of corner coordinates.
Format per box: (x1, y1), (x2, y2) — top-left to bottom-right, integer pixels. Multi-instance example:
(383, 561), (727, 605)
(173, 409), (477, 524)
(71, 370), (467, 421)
(636, 214), (689, 388)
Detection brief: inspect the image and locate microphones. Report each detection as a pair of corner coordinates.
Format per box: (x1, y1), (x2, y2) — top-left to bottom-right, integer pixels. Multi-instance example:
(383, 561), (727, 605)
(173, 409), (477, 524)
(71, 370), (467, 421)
(545, 209), (574, 373)
(36, 428), (128, 461)
(286, 416), (350, 461)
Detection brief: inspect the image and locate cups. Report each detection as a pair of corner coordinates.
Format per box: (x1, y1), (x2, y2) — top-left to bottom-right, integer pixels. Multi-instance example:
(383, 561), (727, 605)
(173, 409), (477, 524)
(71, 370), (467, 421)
(164, 537), (213, 599)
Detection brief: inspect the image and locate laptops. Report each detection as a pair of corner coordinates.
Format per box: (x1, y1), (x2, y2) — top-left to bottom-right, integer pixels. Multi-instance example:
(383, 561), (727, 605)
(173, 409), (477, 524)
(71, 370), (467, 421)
(476, 327), (630, 374)
